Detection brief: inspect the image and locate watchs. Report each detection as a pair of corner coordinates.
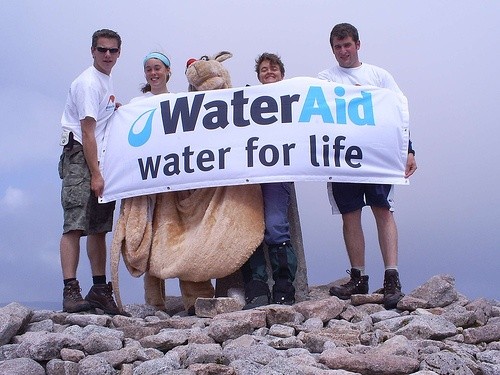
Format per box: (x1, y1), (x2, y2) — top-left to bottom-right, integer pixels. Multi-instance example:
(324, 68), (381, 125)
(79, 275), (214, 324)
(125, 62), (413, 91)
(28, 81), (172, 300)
(408, 150), (415, 156)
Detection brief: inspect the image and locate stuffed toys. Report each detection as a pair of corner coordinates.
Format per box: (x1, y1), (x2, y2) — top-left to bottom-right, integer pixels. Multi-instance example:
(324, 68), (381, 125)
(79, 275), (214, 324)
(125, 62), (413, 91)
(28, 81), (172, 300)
(111, 49), (243, 309)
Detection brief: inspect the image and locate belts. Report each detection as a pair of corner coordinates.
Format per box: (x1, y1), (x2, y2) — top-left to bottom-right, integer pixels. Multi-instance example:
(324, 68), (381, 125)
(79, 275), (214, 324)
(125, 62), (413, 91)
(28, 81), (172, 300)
(64, 141), (83, 157)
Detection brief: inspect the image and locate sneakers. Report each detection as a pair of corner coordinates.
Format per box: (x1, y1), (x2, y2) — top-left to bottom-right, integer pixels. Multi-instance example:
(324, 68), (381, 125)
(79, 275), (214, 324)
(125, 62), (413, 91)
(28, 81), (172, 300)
(63, 280), (89, 312)
(330, 269), (368, 300)
(85, 282), (131, 317)
(242, 295), (272, 309)
(273, 299), (294, 305)
(381, 269), (401, 309)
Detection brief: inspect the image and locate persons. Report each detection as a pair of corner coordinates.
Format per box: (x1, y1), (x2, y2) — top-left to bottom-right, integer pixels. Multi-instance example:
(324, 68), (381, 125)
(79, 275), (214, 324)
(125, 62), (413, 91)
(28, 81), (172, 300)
(58, 29), (134, 317)
(241, 52), (297, 310)
(113, 52), (213, 311)
(310, 23), (417, 306)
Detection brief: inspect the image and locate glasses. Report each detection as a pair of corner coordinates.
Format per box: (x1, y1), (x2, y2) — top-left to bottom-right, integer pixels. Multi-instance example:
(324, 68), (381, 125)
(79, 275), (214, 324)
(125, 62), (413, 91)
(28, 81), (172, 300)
(96, 47), (118, 53)
